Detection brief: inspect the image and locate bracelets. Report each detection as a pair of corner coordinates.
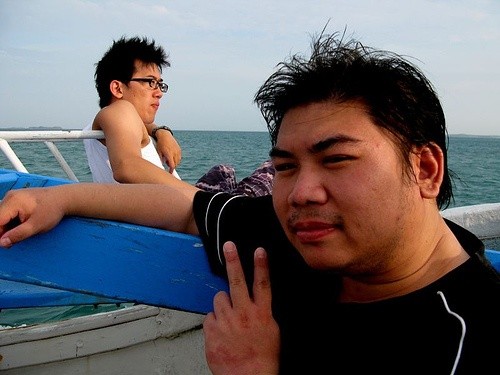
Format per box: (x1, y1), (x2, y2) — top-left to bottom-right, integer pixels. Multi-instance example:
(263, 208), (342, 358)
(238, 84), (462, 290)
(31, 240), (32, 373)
(151, 126), (174, 141)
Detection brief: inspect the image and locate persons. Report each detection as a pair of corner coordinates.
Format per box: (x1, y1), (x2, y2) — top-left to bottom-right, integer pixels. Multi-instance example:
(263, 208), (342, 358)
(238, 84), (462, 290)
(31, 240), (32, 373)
(0, 17), (500, 374)
(79, 33), (275, 212)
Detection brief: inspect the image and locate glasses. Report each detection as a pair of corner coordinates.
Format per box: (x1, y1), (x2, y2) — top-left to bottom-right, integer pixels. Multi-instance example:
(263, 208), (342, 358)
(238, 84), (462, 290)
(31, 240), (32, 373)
(130, 78), (169, 92)
